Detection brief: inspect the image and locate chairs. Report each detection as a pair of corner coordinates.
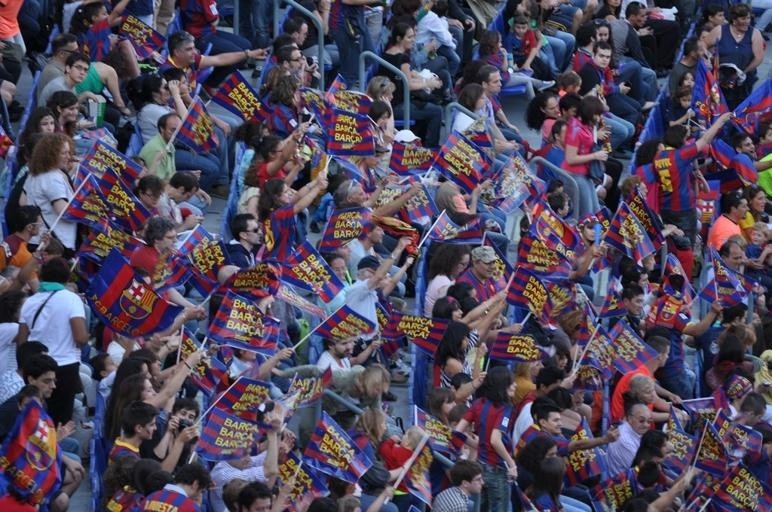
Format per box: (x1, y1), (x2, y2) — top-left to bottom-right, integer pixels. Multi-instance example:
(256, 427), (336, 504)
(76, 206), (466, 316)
(0, 1), (771, 512)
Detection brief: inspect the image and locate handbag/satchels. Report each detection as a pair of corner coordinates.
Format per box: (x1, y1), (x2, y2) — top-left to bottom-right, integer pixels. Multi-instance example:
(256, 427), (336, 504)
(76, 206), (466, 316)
(589, 144), (603, 185)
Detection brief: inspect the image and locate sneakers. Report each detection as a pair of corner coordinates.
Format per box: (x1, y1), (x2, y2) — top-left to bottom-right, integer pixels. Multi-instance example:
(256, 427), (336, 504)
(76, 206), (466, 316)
(210, 186), (230, 200)
(538, 80), (555, 91)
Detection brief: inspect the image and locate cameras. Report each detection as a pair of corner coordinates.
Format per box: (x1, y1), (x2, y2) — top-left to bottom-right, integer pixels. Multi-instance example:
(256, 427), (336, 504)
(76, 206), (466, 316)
(179, 418), (193, 432)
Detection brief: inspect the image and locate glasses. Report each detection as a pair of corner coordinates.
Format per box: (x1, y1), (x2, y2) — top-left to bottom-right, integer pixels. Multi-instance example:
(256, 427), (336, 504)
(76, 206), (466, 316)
(244, 226), (260, 233)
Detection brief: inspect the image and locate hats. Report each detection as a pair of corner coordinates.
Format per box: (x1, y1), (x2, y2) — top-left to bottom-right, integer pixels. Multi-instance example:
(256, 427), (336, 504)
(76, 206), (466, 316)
(358, 256), (379, 273)
(395, 129), (421, 143)
(471, 246), (498, 264)
